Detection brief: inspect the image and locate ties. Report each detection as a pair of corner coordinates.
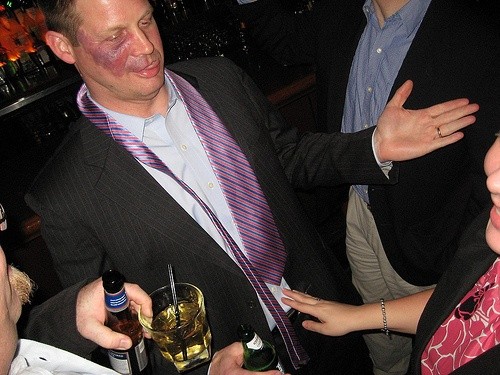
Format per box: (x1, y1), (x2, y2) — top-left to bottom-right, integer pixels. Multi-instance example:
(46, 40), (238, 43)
(76, 63), (310, 370)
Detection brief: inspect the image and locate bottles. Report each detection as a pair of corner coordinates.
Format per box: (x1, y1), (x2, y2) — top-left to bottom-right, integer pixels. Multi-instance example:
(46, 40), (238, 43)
(102, 268), (150, 375)
(0, 24), (65, 104)
(240, 323), (286, 375)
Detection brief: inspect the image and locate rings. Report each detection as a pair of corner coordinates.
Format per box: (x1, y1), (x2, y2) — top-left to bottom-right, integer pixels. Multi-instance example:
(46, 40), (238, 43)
(315, 297), (320, 300)
(436, 127), (443, 138)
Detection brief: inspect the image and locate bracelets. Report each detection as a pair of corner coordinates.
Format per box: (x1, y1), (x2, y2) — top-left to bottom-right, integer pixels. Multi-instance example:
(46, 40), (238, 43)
(379, 299), (390, 334)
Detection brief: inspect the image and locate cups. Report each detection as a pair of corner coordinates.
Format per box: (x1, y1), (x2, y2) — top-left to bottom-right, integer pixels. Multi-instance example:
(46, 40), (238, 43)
(153, 0), (255, 62)
(138, 283), (212, 373)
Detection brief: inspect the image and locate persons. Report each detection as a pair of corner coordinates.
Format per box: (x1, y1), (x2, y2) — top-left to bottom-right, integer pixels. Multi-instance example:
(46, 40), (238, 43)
(0, 0), (479, 375)
(223, 0), (500, 375)
(282, 129), (500, 375)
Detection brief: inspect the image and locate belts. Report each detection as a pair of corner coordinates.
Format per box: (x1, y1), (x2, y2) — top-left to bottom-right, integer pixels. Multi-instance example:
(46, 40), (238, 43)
(274, 308), (298, 339)
